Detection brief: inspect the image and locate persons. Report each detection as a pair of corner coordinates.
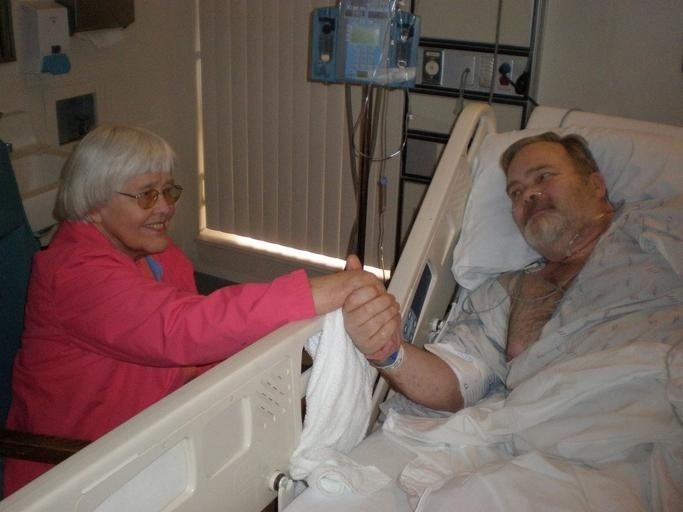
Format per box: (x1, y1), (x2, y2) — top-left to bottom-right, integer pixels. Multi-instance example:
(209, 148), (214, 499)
(336, 132), (679, 510)
(1, 121), (384, 498)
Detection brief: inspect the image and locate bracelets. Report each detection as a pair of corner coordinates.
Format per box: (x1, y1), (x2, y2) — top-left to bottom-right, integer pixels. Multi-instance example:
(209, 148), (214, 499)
(366, 343), (403, 371)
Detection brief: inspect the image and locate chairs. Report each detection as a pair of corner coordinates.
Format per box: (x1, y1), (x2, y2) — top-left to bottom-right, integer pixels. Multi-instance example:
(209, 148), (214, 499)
(0, 141), (94, 466)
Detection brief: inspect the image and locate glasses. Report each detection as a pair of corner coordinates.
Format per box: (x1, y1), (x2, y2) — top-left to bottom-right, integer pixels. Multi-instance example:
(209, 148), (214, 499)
(119, 185), (183, 208)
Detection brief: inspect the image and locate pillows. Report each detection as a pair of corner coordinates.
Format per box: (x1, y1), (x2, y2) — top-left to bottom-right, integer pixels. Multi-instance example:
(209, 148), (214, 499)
(450, 130), (683, 291)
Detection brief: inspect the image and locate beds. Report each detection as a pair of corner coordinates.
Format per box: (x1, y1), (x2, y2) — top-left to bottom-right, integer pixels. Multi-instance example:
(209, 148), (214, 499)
(1, 101), (683, 512)
